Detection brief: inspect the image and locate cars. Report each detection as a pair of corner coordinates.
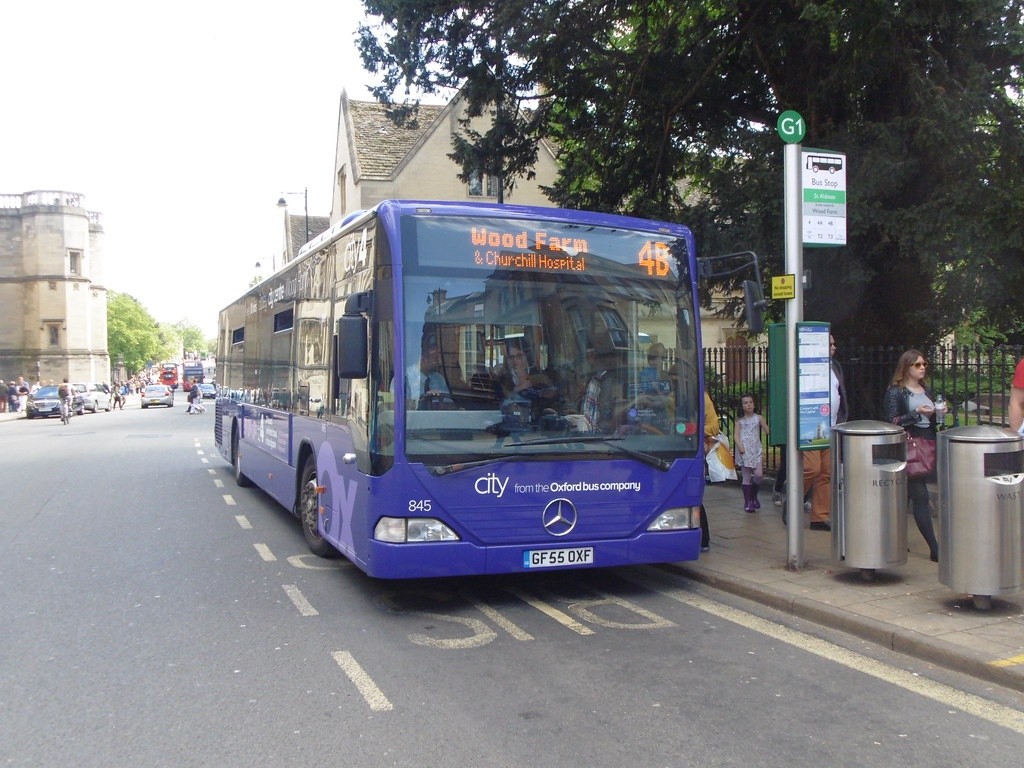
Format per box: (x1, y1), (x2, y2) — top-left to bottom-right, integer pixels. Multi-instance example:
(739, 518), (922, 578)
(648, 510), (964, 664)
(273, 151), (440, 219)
(72, 382), (112, 413)
(141, 385), (172, 408)
(201, 384), (216, 399)
(167, 386), (175, 400)
(26, 384), (85, 419)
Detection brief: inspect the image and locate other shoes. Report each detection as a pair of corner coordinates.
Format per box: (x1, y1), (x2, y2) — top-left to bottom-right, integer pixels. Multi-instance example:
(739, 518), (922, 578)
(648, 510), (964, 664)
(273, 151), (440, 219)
(782, 500), (787, 524)
(61, 418), (64, 421)
(771, 494), (782, 506)
(804, 502), (811, 513)
(810, 522), (831, 530)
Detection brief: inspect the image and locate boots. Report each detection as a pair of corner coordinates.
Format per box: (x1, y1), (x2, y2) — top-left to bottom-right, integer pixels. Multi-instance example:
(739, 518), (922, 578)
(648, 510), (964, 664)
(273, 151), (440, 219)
(742, 484), (754, 512)
(752, 484), (760, 508)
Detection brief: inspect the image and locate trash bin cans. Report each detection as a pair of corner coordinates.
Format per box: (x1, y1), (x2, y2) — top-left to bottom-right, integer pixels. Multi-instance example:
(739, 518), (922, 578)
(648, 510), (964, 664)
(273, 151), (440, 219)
(936, 425), (1024, 609)
(829, 419), (907, 581)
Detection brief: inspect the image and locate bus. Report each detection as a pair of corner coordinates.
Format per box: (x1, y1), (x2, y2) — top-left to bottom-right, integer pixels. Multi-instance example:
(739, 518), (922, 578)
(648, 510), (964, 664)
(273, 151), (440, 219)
(160, 370), (177, 388)
(183, 361), (205, 392)
(162, 363), (179, 386)
(214, 198), (764, 578)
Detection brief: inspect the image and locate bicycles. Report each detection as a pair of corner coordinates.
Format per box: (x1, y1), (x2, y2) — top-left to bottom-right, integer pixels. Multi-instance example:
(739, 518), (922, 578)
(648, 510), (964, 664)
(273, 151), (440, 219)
(62, 398), (71, 425)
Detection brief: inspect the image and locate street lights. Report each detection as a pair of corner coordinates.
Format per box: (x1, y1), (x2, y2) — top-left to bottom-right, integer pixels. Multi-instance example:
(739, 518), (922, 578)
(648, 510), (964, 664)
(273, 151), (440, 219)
(255, 262), (276, 274)
(277, 191), (309, 243)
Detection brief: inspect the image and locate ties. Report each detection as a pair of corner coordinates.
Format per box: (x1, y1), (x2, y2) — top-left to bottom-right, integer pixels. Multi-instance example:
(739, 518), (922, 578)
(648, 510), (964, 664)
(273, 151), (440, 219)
(424, 378), (430, 404)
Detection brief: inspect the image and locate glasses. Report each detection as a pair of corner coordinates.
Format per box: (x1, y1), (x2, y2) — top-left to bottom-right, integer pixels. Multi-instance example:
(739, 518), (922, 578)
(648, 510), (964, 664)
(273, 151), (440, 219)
(507, 352), (525, 361)
(908, 361), (928, 369)
(829, 343), (837, 347)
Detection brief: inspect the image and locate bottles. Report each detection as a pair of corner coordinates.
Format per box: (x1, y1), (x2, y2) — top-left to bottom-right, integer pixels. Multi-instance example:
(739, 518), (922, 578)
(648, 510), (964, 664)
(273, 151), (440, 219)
(935, 394), (946, 423)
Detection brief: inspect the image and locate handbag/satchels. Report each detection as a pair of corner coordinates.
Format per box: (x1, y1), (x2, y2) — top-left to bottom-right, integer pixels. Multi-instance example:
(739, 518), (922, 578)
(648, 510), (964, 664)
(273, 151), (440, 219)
(704, 430), (738, 482)
(11, 394), (17, 401)
(903, 434), (936, 476)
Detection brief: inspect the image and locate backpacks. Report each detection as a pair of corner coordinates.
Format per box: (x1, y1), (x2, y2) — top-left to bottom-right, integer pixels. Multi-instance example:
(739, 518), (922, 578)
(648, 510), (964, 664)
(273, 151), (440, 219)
(59, 385), (69, 398)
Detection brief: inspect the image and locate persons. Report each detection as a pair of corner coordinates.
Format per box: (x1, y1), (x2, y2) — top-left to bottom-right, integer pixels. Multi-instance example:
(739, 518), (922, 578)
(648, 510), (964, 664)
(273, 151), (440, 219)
(1007, 359), (1024, 433)
(963, 392), (990, 412)
(446, 361), (468, 411)
(315, 395), (327, 418)
(564, 348), (616, 435)
(670, 365), (719, 552)
(103, 381), (111, 392)
(113, 379), (127, 410)
(185, 378), (206, 415)
(389, 344), (454, 411)
(641, 344), (670, 434)
(885, 350), (948, 563)
(773, 335), (849, 531)
(490, 336), (540, 436)
(126, 370), (152, 395)
(223, 385), (289, 413)
(58, 378), (76, 421)
(0, 376), (54, 415)
(734, 394), (769, 513)
